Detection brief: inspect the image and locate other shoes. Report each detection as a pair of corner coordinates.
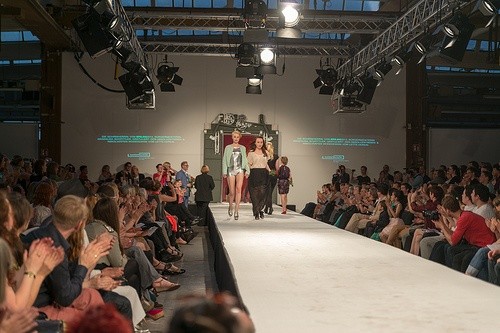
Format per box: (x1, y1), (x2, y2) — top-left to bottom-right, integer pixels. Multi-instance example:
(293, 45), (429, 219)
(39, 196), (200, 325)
(189, 216), (204, 225)
(153, 301), (163, 308)
(181, 228), (199, 243)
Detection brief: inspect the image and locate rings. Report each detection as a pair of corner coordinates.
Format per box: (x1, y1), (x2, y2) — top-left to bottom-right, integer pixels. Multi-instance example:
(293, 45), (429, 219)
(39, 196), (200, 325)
(94, 254), (98, 258)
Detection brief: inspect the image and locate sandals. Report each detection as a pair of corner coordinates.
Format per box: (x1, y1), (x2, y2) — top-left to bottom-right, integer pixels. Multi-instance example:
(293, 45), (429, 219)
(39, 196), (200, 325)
(152, 278), (181, 293)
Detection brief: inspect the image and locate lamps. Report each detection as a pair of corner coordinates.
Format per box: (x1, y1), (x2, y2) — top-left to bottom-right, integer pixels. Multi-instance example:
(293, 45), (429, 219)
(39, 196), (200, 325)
(72, 13), (113, 60)
(313, 0), (500, 113)
(232, 34), (278, 95)
(155, 53), (183, 94)
(101, 12), (155, 108)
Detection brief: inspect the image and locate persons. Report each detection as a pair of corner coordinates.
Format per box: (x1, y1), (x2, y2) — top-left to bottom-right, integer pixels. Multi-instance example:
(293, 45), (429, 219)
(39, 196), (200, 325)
(299, 159), (500, 287)
(0, 154), (195, 333)
(221, 131), (251, 220)
(265, 141), (279, 215)
(243, 143), (252, 203)
(171, 296), (256, 333)
(195, 165), (215, 226)
(277, 156), (294, 214)
(247, 136), (271, 220)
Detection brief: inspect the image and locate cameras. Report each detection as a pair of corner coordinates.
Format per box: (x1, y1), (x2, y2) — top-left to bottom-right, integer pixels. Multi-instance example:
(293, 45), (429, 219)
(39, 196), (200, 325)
(415, 191), (421, 196)
(422, 209), (441, 221)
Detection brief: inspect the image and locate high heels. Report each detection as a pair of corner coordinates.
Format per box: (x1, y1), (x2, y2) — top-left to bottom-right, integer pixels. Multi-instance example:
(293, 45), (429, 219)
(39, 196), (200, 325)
(166, 246), (183, 260)
(281, 210), (286, 214)
(228, 207), (233, 216)
(268, 208), (273, 215)
(234, 211), (239, 220)
(146, 308), (164, 319)
(172, 265), (185, 274)
(152, 262), (172, 271)
(264, 207), (267, 213)
(260, 211), (264, 218)
(255, 213), (259, 220)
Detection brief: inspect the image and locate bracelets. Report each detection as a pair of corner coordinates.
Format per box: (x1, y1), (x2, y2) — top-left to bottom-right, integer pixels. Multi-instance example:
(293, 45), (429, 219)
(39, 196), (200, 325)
(23, 271), (36, 278)
